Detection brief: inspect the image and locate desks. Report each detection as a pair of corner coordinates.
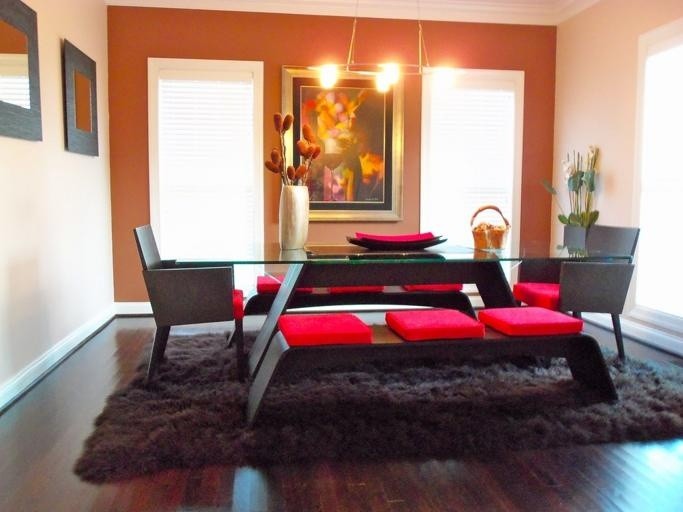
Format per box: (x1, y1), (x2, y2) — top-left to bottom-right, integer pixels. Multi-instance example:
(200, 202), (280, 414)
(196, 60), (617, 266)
(175, 231), (633, 377)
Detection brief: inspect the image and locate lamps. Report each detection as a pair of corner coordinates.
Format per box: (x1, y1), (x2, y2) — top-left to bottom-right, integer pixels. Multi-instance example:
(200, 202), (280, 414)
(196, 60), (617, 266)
(306, 16), (436, 95)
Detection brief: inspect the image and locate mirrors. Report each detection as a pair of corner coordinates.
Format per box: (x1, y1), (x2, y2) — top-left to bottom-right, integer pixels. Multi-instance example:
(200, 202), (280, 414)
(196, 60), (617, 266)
(63, 37), (99, 157)
(0, 1), (43, 142)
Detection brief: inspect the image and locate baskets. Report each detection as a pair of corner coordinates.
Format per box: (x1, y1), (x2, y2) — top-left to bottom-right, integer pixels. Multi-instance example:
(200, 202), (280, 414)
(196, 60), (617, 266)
(471, 205), (510, 248)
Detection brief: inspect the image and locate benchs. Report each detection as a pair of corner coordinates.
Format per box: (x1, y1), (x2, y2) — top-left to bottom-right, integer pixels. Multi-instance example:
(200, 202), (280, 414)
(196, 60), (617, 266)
(245, 311), (619, 431)
(225, 275), (481, 351)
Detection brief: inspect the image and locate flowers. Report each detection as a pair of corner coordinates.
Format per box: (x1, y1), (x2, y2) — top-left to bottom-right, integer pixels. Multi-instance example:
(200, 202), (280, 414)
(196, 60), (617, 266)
(542, 143), (600, 225)
(265, 108), (321, 186)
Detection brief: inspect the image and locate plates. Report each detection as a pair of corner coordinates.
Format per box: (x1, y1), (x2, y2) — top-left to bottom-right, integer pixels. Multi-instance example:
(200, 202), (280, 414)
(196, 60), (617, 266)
(345, 236), (447, 250)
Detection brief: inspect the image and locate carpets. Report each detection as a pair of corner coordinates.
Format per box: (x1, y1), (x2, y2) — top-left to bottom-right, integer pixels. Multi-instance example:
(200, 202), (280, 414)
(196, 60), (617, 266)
(72, 331), (683, 482)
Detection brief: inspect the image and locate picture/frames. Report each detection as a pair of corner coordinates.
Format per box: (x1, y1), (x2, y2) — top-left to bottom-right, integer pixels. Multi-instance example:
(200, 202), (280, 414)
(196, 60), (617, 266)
(281, 62), (404, 223)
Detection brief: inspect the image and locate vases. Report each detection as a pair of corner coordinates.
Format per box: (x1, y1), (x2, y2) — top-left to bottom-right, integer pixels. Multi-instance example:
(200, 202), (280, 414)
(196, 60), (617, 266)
(277, 186), (311, 252)
(561, 226), (588, 252)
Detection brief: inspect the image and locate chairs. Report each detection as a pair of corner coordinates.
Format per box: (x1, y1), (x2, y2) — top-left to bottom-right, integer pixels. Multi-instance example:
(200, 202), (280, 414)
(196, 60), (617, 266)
(133, 222), (246, 391)
(512, 223), (641, 363)
